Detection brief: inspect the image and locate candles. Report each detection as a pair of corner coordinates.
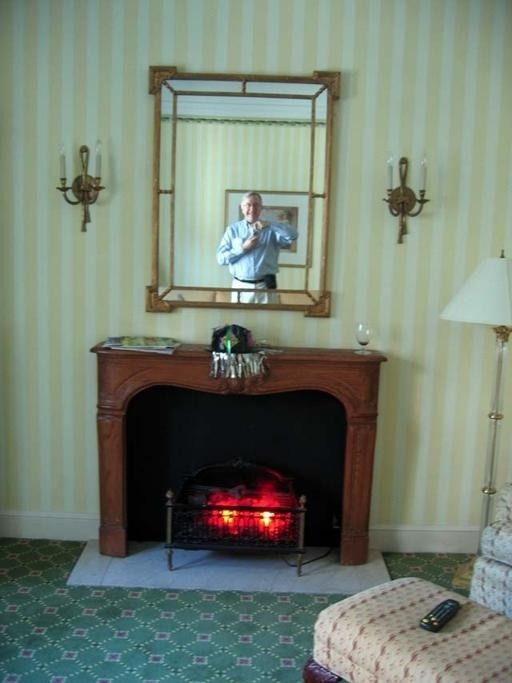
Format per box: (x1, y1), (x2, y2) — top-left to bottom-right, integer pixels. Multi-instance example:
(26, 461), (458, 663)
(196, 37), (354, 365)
(385, 152), (428, 190)
(57, 135), (103, 178)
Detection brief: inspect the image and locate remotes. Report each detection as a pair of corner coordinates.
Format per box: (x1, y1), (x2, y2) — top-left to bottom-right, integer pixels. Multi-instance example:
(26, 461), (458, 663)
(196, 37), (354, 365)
(420, 599), (458, 632)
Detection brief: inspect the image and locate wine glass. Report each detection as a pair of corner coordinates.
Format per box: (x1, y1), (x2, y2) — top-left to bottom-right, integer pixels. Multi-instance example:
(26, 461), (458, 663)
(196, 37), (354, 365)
(354, 319), (375, 357)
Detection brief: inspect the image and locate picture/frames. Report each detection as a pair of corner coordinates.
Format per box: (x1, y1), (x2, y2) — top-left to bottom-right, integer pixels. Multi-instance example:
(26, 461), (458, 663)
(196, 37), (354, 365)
(224, 188), (314, 268)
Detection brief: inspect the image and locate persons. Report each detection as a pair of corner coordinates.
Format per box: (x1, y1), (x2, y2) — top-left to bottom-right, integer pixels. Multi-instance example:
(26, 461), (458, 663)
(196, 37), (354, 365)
(216, 192), (298, 304)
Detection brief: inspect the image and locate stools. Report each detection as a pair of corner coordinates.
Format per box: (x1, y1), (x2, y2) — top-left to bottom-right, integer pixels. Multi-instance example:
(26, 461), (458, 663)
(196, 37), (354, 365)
(303, 577), (511, 682)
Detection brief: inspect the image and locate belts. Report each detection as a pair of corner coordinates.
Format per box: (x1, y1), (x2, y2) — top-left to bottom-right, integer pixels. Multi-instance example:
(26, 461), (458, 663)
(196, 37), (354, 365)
(234, 276), (263, 284)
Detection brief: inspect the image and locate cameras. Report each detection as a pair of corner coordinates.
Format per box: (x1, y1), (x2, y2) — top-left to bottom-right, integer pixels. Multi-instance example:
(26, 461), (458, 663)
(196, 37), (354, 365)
(252, 228), (258, 237)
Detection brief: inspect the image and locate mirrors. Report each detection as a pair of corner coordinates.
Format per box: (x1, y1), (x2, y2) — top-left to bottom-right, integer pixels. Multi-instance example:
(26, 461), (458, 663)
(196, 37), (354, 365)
(147, 62), (342, 318)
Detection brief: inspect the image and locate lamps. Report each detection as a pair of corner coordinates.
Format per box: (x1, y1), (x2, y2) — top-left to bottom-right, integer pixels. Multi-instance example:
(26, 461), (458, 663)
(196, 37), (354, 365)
(439, 248), (512, 559)
